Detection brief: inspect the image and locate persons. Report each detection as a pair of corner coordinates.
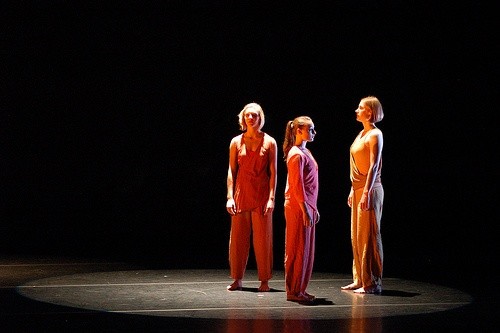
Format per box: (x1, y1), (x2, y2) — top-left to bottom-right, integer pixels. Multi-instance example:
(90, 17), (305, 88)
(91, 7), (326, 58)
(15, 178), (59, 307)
(341, 97), (385, 293)
(226, 103), (277, 292)
(283, 116), (320, 302)
